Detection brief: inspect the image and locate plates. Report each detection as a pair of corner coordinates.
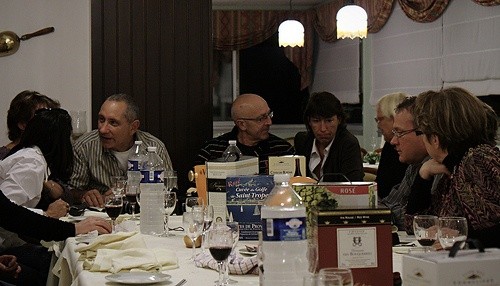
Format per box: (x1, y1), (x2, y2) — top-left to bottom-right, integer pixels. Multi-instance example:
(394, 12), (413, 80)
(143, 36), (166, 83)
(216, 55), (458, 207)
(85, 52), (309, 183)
(238, 245), (257, 255)
(105, 272), (172, 285)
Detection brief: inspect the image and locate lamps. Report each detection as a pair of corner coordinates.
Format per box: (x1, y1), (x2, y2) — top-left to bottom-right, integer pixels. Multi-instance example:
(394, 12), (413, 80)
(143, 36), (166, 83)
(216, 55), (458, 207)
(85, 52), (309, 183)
(277, 0), (305, 48)
(335, 0), (368, 40)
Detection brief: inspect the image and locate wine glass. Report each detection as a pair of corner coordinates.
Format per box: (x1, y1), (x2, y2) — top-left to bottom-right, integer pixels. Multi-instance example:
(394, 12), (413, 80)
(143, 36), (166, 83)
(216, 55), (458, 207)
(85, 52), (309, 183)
(159, 192), (177, 237)
(104, 176), (140, 234)
(182, 197), (240, 286)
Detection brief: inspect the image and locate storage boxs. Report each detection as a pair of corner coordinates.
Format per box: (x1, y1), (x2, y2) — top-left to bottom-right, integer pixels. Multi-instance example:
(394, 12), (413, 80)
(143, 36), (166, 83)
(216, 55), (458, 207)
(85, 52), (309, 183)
(205, 154), (260, 227)
(269, 155), (307, 191)
(226, 175), (275, 241)
(393, 247), (500, 286)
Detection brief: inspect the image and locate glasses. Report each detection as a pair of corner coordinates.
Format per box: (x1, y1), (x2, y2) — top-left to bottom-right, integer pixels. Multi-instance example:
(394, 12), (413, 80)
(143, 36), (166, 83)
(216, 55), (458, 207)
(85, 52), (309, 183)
(392, 126), (420, 139)
(239, 110), (273, 123)
(375, 116), (389, 123)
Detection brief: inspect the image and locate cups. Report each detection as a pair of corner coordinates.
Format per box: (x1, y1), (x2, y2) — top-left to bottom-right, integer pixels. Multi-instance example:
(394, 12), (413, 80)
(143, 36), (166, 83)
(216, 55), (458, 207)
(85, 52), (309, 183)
(163, 171), (177, 192)
(437, 217), (467, 250)
(293, 244), (354, 286)
(413, 215), (438, 246)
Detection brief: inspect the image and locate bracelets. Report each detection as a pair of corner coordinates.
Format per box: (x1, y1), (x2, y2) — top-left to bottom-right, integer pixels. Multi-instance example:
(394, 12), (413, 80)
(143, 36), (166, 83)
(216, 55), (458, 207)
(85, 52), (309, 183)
(48, 181), (54, 194)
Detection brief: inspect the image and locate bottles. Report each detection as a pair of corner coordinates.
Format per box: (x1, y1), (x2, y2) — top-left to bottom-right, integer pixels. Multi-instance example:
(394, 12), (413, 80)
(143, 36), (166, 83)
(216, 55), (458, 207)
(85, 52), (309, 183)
(292, 158), (302, 177)
(139, 146), (164, 236)
(223, 140), (242, 162)
(127, 141), (146, 192)
(262, 174), (307, 286)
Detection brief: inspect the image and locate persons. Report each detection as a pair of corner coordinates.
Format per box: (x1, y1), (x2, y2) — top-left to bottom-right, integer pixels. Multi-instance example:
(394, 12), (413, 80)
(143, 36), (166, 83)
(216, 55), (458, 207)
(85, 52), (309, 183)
(0, 89), (72, 211)
(197, 93), (295, 192)
(0, 189), (111, 286)
(0, 108), (74, 286)
(403, 85), (500, 250)
(373, 92), (408, 199)
(378, 95), (444, 230)
(294, 92), (365, 183)
(67, 93), (173, 209)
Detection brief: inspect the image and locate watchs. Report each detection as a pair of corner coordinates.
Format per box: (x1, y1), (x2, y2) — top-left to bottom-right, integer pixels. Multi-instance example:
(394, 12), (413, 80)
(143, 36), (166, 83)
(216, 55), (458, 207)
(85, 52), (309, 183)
(44, 212), (48, 217)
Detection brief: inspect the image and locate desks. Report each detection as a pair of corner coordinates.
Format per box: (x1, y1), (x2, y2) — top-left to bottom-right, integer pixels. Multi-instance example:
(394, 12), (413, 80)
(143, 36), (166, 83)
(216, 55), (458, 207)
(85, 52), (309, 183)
(41, 189), (438, 286)
